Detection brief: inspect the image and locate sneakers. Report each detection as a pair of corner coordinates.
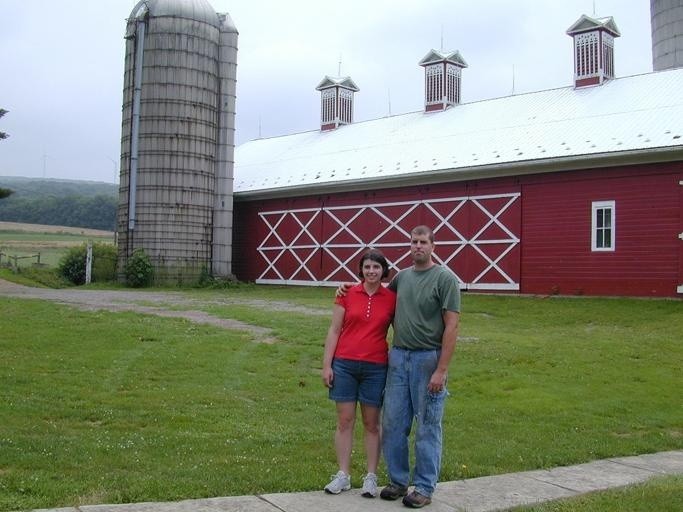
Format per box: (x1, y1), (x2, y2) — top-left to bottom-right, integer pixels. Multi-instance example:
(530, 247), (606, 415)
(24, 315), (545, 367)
(380, 484), (408, 500)
(402, 491), (431, 508)
(324, 471), (351, 494)
(360, 473), (377, 497)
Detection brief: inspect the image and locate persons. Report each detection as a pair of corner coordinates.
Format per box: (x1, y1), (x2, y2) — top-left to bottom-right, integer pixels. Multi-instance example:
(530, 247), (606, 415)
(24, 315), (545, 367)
(320, 249), (396, 497)
(335, 224), (462, 508)
